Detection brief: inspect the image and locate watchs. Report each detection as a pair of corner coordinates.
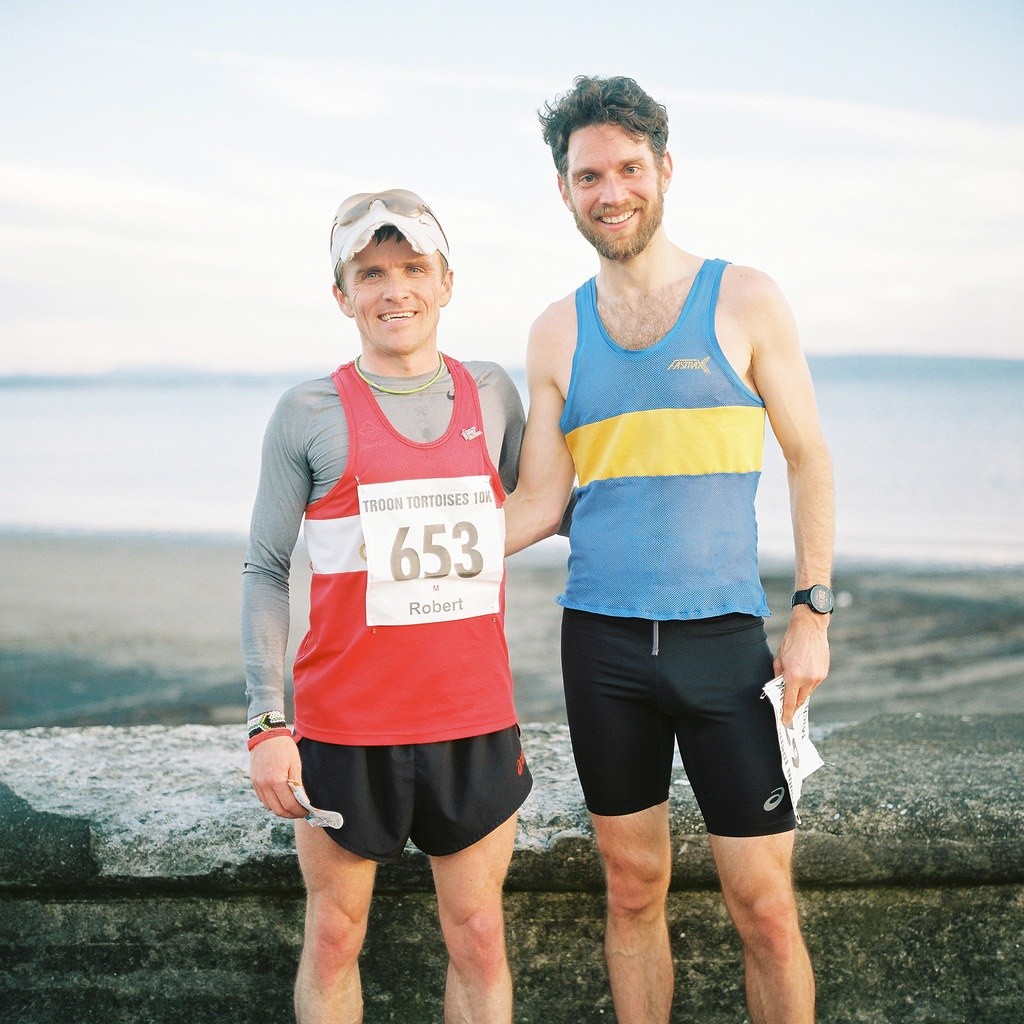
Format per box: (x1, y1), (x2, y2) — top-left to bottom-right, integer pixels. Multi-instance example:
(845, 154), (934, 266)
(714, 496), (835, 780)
(791, 585), (836, 614)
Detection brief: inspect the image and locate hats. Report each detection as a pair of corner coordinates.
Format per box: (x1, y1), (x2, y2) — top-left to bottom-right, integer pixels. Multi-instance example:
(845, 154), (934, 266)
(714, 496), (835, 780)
(329, 202), (452, 273)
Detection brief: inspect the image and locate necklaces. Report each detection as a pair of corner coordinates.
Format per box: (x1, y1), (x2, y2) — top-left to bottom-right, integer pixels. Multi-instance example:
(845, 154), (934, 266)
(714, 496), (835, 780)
(354, 350), (444, 394)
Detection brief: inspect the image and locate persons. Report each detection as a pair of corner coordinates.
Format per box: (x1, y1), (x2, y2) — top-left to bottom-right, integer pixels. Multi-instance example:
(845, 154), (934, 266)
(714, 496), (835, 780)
(504, 78), (835, 1024)
(240, 200), (578, 1024)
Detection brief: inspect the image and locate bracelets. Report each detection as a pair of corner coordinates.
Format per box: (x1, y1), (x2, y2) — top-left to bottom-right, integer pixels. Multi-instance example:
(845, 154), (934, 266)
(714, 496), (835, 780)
(247, 712), (292, 750)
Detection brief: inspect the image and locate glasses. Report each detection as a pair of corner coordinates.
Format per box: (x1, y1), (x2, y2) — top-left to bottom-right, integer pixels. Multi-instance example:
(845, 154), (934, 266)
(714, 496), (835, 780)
(330, 189), (452, 252)
